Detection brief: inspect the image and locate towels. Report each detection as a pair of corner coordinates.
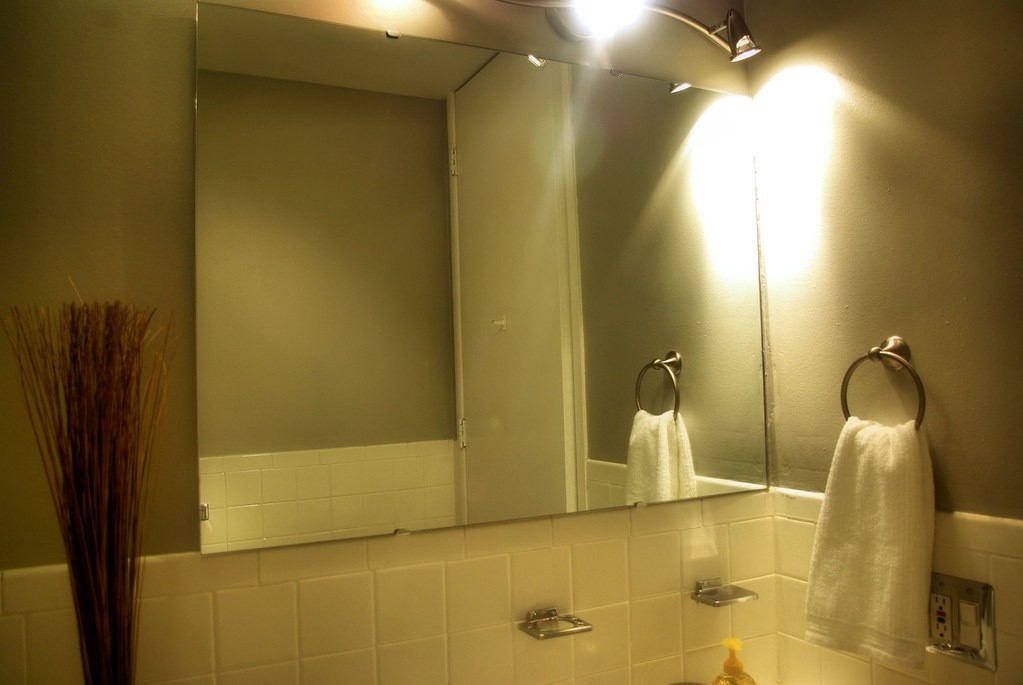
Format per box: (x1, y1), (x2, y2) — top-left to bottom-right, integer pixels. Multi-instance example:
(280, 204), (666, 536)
(625, 409), (694, 505)
(803, 415), (933, 671)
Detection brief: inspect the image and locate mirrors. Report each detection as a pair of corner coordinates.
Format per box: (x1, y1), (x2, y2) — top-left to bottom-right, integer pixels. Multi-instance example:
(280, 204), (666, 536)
(196, 0), (770, 554)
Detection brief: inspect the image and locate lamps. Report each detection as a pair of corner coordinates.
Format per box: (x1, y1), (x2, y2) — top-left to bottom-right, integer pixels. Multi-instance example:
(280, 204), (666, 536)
(708, 9), (763, 62)
(669, 82), (693, 93)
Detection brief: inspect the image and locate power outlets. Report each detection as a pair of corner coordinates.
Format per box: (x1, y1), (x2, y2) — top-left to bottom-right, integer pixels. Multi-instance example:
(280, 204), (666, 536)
(924, 571), (1000, 673)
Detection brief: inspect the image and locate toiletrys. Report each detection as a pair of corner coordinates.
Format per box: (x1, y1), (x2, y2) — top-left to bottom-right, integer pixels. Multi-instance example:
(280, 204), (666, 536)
(709, 637), (757, 685)
